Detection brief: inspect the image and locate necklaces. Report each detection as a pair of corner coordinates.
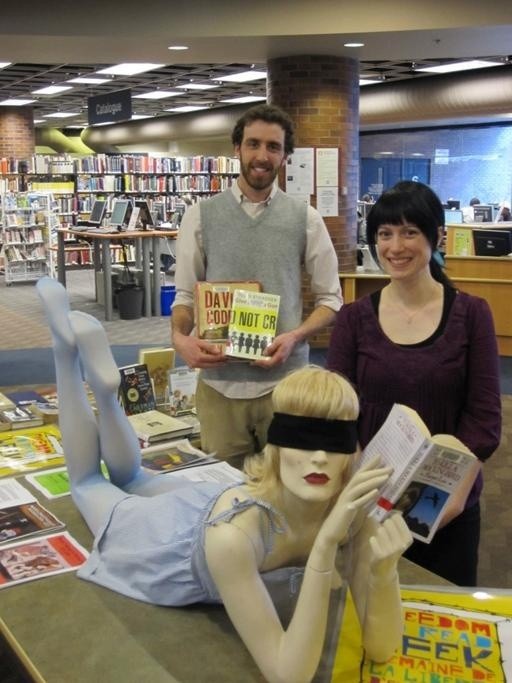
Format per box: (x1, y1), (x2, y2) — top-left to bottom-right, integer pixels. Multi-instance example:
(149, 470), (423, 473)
(389, 286), (435, 326)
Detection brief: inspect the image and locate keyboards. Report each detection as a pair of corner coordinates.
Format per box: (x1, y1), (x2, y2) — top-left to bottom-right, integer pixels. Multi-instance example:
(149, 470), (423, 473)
(71, 226), (94, 232)
(88, 228), (119, 234)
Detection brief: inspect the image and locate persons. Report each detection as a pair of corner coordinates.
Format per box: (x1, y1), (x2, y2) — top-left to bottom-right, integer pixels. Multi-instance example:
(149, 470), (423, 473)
(37, 275), (413, 683)
(328, 182), (501, 586)
(171, 105), (344, 469)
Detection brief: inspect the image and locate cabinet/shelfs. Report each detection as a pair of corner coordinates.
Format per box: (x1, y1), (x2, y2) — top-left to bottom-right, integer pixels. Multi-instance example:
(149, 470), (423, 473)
(2, 191), (55, 281)
(0, 172), (238, 267)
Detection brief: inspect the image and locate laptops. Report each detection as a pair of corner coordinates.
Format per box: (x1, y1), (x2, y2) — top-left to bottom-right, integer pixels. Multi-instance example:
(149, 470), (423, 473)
(77, 200), (108, 226)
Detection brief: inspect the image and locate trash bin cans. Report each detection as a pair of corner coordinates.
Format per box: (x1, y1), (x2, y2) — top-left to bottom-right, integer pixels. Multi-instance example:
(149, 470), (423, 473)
(114, 284), (145, 319)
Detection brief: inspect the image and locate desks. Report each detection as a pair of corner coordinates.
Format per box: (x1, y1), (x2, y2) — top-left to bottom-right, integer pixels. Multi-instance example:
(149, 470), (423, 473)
(54, 227), (178, 321)
(0, 438), (457, 682)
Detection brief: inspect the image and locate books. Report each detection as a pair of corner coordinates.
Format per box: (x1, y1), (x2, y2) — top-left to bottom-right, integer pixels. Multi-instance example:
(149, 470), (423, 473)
(357, 403), (477, 544)
(0, 348), (219, 476)
(223, 289), (281, 365)
(1, 157), (244, 265)
(193, 279), (260, 362)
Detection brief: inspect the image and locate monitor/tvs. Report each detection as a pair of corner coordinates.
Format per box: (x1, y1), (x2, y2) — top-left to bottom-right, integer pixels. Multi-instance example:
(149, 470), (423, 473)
(124, 201), (133, 225)
(444, 209), (462, 224)
(472, 230), (512, 256)
(109, 200), (129, 232)
(135, 201), (155, 231)
(447, 200), (460, 209)
(473, 204), (494, 224)
(151, 202), (166, 226)
(170, 203), (187, 230)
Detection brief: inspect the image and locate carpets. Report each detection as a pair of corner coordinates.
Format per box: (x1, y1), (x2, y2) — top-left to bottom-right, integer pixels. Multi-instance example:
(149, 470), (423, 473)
(1, 343), (511, 396)
